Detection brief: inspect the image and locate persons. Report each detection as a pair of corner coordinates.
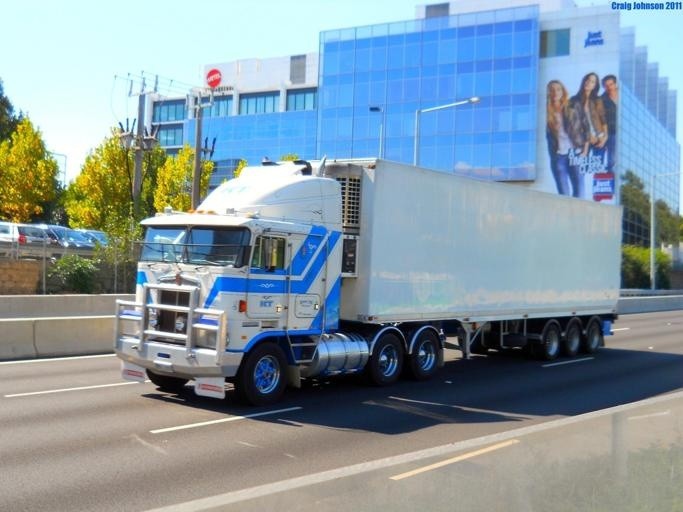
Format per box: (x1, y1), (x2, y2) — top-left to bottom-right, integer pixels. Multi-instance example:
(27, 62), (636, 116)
(569, 72), (607, 172)
(598, 76), (618, 195)
(547, 79), (590, 198)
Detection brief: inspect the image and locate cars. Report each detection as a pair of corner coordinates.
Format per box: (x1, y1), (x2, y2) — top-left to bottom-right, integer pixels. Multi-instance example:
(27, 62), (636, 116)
(0, 220), (110, 266)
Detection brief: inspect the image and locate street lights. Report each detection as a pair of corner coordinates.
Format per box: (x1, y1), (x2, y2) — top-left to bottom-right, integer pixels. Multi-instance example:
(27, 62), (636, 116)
(368, 105), (385, 157)
(412, 97), (484, 169)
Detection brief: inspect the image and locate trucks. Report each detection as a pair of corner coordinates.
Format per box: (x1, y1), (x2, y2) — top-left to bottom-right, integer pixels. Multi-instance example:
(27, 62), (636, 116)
(110, 156), (630, 400)
